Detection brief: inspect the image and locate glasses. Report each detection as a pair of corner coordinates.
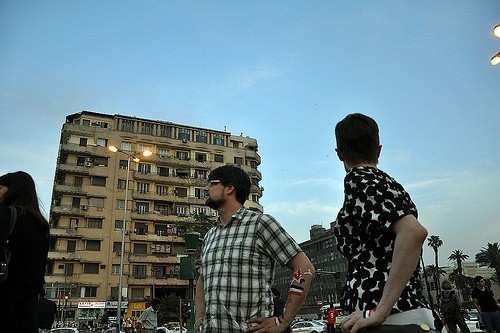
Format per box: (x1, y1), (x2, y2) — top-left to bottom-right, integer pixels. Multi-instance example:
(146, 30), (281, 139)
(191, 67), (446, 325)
(209, 179), (220, 187)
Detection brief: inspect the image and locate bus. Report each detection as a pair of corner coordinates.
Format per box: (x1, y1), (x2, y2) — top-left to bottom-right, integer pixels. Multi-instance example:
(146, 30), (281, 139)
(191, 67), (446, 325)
(319, 302), (347, 327)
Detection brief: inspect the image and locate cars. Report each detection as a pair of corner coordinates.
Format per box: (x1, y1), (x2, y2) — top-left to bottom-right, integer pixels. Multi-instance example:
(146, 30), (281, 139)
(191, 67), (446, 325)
(290, 320), (336, 333)
(157, 322), (188, 333)
(50, 327), (81, 333)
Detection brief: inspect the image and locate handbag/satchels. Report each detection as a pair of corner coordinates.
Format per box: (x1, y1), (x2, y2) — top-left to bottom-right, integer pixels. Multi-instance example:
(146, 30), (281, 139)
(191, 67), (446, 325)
(0, 206), (17, 278)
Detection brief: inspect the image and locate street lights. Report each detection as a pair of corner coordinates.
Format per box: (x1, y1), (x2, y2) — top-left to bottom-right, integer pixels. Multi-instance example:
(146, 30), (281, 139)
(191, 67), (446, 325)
(64, 295), (69, 322)
(107, 145), (153, 333)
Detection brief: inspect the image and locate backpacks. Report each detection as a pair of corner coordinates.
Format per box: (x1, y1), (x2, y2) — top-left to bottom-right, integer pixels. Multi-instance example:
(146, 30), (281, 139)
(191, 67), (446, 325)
(440, 290), (454, 314)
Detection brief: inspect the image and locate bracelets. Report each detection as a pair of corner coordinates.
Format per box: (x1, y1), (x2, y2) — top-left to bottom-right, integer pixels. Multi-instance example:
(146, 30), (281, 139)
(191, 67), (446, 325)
(195, 318), (203, 325)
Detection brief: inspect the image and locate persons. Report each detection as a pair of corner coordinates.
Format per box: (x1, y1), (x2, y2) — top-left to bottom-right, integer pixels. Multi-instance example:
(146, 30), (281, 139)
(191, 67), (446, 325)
(137, 298), (161, 333)
(472, 276), (500, 333)
(437, 280), (471, 333)
(333, 113), (435, 333)
(194, 166), (315, 333)
(0, 172), (50, 333)
(327, 305), (338, 333)
